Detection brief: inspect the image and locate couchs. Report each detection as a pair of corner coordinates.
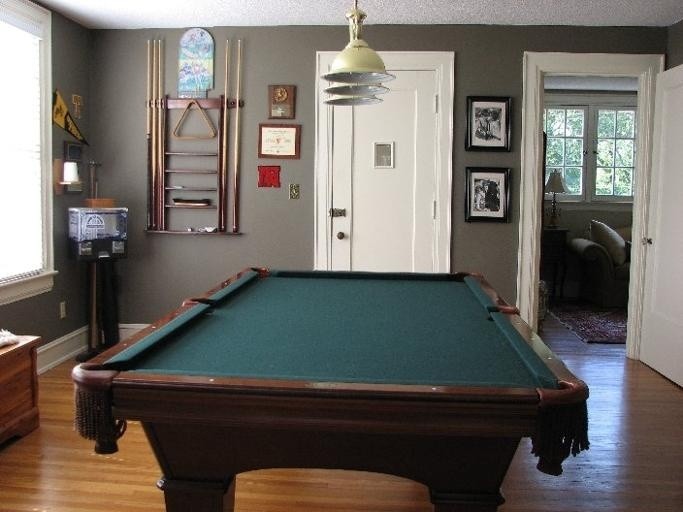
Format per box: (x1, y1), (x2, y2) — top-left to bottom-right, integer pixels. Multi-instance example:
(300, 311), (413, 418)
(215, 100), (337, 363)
(569, 236), (628, 314)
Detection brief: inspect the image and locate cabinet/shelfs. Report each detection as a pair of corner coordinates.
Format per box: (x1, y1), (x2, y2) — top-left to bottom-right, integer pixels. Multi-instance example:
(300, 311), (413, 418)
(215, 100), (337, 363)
(0, 332), (45, 446)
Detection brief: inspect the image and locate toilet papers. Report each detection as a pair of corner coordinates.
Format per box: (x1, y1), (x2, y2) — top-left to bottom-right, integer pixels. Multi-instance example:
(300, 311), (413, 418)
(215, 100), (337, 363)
(62, 162), (81, 183)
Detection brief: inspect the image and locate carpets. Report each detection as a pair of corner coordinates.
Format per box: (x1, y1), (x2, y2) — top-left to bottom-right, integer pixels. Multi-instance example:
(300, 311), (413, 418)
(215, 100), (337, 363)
(545, 303), (628, 347)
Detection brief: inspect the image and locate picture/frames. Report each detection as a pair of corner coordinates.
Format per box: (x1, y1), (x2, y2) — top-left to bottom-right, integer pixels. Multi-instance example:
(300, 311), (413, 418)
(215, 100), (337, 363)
(464, 165), (510, 224)
(63, 140), (83, 163)
(465, 94), (512, 153)
(257, 123), (301, 160)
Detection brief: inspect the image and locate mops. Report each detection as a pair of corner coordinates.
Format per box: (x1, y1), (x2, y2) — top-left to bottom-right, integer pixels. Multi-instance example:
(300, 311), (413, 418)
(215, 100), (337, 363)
(74, 262), (99, 364)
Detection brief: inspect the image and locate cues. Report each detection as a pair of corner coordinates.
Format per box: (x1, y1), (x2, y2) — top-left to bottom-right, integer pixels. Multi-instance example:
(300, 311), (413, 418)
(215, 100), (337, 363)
(221, 39), (228, 231)
(232, 39), (240, 231)
(146, 39), (164, 229)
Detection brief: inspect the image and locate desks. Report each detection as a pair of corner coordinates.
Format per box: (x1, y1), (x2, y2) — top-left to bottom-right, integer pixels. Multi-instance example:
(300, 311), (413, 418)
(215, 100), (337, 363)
(541, 226), (570, 305)
(71, 239), (130, 363)
(69, 264), (590, 511)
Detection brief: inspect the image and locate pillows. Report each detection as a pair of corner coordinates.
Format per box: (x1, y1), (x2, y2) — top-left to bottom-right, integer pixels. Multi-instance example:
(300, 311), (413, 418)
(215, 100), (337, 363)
(588, 217), (626, 268)
(623, 236), (632, 257)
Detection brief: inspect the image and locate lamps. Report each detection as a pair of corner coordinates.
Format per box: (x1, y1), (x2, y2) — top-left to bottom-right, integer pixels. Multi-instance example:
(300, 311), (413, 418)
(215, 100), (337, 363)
(543, 167), (564, 228)
(318, 0), (397, 84)
(320, 92), (384, 108)
(321, 80), (392, 98)
